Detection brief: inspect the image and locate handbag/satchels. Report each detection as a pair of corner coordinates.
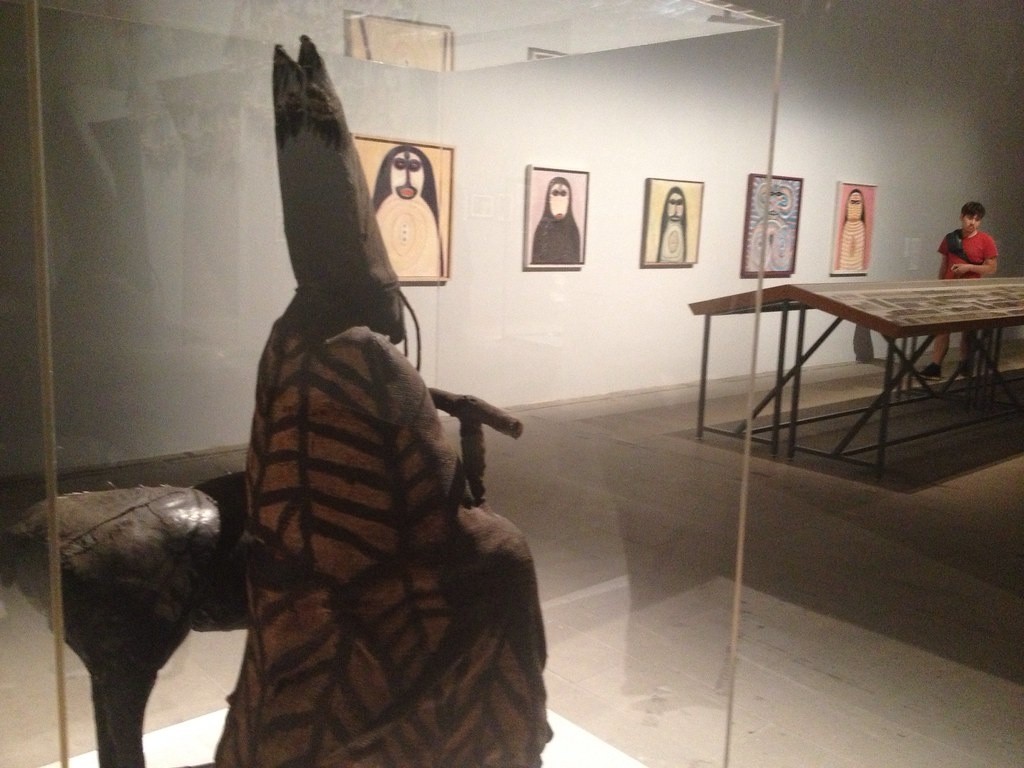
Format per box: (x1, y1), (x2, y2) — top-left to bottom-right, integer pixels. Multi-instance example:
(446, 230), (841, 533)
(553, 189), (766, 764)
(945, 229), (983, 265)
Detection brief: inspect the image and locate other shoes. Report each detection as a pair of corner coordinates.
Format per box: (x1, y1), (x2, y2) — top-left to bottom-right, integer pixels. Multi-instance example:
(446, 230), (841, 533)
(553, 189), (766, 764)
(957, 359), (974, 377)
(919, 363), (941, 381)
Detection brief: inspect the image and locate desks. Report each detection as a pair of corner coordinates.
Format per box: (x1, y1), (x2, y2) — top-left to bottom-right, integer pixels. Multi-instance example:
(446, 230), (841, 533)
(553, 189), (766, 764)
(688, 275), (1024, 481)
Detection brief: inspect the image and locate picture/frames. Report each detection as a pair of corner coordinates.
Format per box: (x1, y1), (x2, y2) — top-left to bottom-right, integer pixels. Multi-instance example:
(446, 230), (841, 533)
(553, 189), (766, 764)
(522, 164), (590, 271)
(638, 178), (705, 267)
(741, 173), (805, 278)
(349, 132), (456, 281)
(828, 179), (878, 277)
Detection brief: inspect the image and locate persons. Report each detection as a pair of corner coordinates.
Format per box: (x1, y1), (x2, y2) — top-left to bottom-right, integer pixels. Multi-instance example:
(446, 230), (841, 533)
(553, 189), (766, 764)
(918, 201), (998, 382)
